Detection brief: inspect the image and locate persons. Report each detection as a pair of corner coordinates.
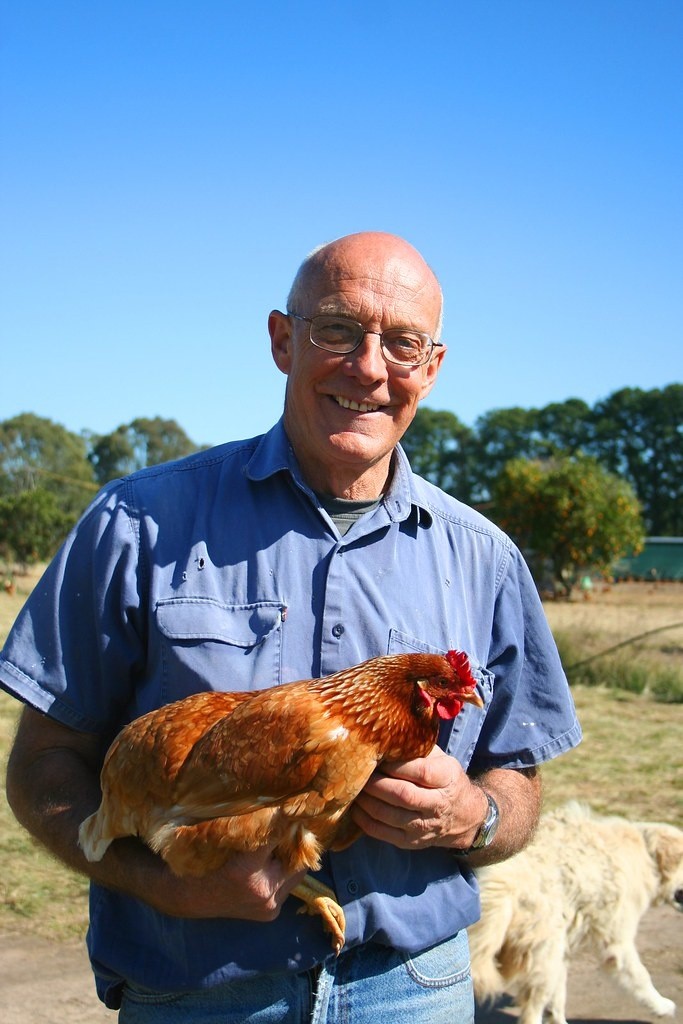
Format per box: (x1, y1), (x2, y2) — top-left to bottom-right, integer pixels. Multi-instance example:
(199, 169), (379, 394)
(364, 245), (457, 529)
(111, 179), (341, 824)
(2, 232), (585, 1024)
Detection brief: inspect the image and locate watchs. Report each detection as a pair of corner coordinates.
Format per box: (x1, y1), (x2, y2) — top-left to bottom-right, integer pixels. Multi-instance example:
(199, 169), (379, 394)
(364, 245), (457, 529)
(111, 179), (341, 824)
(449, 785), (500, 855)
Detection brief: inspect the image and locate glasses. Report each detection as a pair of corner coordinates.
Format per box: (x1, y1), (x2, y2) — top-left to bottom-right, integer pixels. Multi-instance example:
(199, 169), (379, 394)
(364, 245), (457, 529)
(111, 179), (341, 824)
(286, 311), (443, 367)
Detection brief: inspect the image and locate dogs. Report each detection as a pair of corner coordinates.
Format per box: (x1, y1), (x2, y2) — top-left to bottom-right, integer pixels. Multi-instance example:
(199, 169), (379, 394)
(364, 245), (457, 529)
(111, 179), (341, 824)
(467, 801), (683, 1024)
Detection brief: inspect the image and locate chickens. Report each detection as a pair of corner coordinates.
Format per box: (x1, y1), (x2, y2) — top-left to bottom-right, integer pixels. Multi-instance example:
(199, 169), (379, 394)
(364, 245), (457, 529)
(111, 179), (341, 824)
(74, 649), (486, 960)
(4, 580), (17, 597)
(540, 575), (615, 604)
